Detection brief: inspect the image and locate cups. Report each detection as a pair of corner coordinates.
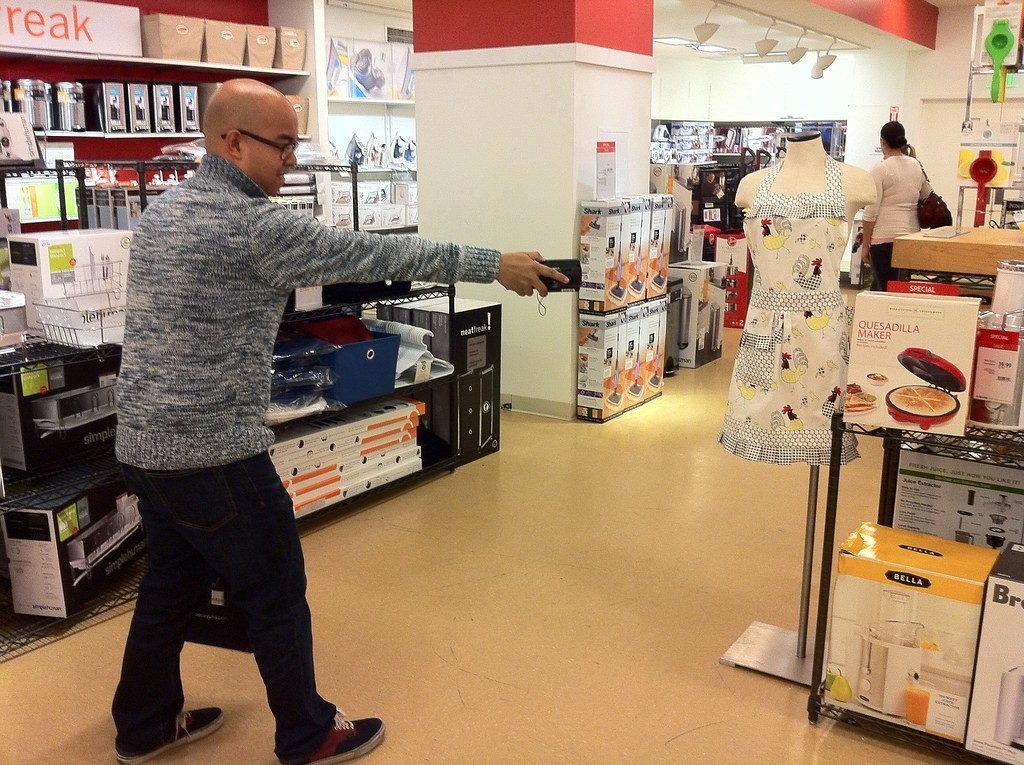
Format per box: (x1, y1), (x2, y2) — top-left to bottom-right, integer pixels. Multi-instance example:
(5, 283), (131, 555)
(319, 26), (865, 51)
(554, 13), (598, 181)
(903, 676), (935, 729)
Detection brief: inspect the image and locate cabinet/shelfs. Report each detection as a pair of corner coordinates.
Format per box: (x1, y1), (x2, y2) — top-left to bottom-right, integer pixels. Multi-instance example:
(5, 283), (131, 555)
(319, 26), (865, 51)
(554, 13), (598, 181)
(649, 116), (845, 161)
(951, 5), (1024, 231)
(802, 409), (1024, 765)
(318, 31), (419, 237)
(0, 261), (460, 655)
(0, 0), (337, 227)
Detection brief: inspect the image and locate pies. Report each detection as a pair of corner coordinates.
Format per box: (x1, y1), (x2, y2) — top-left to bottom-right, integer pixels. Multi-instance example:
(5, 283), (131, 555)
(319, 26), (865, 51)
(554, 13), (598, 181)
(889, 386), (956, 416)
(845, 396), (874, 411)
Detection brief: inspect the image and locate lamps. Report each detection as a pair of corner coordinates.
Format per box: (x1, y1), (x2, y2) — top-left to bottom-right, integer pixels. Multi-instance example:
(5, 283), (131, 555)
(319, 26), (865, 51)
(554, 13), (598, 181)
(692, 2), (724, 44)
(810, 61), (823, 79)
(751, 16), (781, 58)
(817, 38), (838, 72)
(787, 27), (810, 65)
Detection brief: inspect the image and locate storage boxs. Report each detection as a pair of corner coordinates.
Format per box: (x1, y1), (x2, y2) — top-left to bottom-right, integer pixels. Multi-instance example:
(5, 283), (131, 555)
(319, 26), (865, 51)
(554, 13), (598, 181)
(274, 24), (307, 72)
(0, 478), (146, 617)
(953, 120), (1020, 191)
(965, 542), (1024, 765)
(7, 230), (135, 337)
(891, 447), (1024, 549)
(394, 296), (452, 396)
(376, 294), (447, 321)
(331, 179), (419, 232)
(199, 82), (223, 130)
(140, 13), (203, 61)
(323, 33), (416, 101)
(0, 111), (41, 164)
(842, 289), (982, 437)
(414, 298), (478, 431)
(0, 344), (124, 473)
(580, 165), (756, 424)
(0, 0), (143, 57)
(75, 185), (164, 229)
(432, 300), (505, 471)
(823, 522), (1001, 744)
(268, 322), (427, 519)
(201, 18), (246, 66)
(285, 93), (310, 135)
(671, 122), (713, 163)
(245, 22), (276, 68)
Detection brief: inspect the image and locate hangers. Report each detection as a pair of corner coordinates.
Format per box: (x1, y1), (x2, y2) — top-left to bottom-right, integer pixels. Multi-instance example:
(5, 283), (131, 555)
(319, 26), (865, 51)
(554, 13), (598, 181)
(359, 316), (456, 390)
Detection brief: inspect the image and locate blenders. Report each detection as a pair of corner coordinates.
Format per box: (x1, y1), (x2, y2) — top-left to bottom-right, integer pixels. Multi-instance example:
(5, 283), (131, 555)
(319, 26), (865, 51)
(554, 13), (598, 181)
(854, 589), (925, 719)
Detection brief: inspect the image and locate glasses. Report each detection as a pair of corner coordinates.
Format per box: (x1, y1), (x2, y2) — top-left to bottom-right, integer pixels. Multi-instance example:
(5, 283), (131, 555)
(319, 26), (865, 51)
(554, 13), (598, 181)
(220, 129), (301, 160)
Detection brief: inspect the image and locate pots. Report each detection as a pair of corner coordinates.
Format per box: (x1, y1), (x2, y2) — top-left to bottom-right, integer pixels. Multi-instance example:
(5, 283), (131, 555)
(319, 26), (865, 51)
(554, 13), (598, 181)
(885, 347), (967, 429)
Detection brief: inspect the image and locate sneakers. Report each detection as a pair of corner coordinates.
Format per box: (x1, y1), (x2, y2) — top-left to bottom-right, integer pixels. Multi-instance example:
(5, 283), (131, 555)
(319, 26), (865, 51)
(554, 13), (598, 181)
(115, 707), (224, 765)
(275, 706), (386, 765)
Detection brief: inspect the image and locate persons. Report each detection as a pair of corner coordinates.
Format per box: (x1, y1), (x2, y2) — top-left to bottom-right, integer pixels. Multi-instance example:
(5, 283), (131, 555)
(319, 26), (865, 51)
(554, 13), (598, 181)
(112, 78), (569, 765)
(862, 122), (930, 292)
(717, 131), (878, 466)
(703, 172), (722, 197)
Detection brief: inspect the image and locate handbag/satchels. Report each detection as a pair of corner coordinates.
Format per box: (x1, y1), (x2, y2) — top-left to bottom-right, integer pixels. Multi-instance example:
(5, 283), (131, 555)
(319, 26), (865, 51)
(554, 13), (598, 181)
(915, 158), (953, 230)
(183, 572), (257, 654)
